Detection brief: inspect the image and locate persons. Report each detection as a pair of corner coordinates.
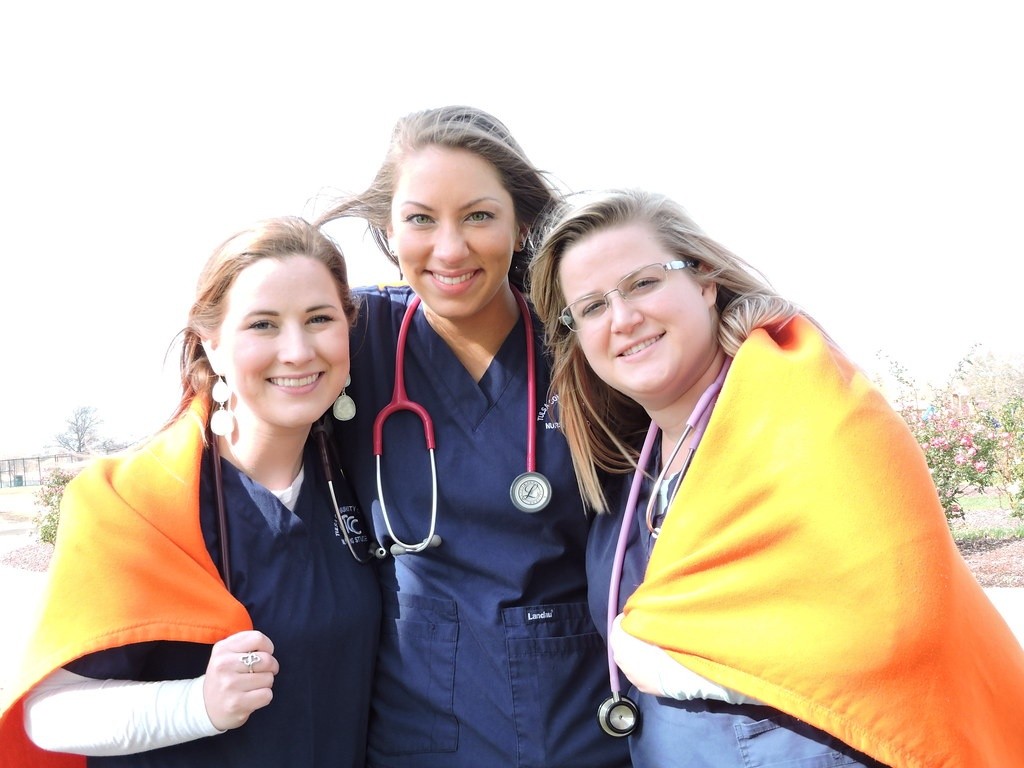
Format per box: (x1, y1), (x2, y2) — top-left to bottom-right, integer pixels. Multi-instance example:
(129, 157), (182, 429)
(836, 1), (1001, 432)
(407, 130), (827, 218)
(333, 107), (633, 767)
(525, 184), (1022, 767)
(23, 216), (378, 767)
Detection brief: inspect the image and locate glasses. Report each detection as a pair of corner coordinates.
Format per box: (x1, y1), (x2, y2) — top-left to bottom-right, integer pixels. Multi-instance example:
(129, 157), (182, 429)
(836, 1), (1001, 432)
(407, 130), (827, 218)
(558, 259), (698, 333)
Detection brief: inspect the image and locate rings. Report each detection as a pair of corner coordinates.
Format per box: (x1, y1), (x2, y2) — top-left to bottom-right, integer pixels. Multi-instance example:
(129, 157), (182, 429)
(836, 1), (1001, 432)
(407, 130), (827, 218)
(239, 651), (259, 674)
